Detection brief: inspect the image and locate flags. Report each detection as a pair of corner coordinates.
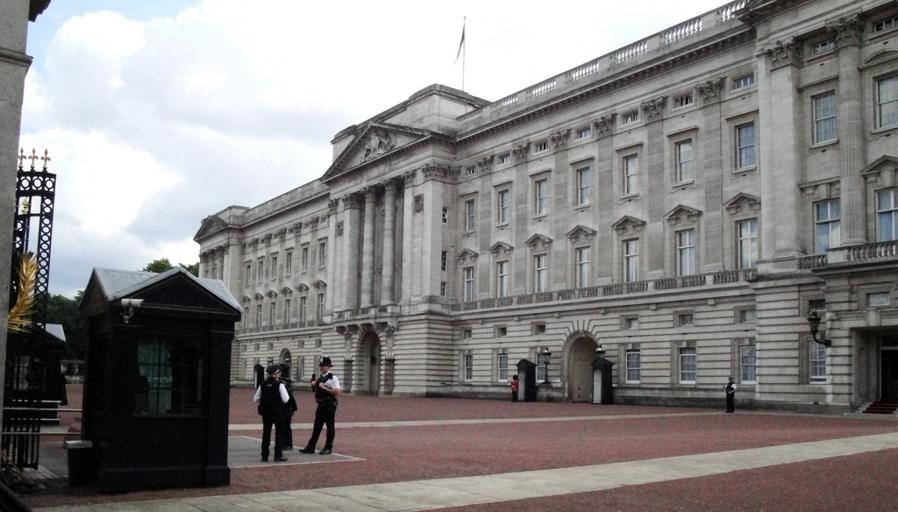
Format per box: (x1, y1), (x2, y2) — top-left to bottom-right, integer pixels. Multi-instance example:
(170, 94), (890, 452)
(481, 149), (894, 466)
(453, 24), (466, 66)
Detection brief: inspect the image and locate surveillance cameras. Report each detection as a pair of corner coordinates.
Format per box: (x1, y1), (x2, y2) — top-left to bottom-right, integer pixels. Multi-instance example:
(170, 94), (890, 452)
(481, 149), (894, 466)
(120, 298), (144, 308)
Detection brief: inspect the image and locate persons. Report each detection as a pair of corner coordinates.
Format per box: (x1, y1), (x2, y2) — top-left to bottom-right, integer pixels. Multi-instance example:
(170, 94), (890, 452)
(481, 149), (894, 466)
(297, 355), (341, 455)
(276, 363), (298, 450)
(723, 374), (738, 413)
(252, 363), (290, 462)
(309, 373), (317, 392)
(510, 374), (519, 402)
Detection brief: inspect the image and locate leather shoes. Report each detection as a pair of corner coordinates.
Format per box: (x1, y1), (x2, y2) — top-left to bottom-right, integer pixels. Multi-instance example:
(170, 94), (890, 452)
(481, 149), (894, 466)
(274, 457), (288, 462)
(319, 449), (332, 455)
(261, 457), (267, 462)
(299, 448), (314, 453)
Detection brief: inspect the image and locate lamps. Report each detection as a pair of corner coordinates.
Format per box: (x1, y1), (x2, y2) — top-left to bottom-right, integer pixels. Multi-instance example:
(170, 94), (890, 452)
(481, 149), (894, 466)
(805, 311), (831, 349)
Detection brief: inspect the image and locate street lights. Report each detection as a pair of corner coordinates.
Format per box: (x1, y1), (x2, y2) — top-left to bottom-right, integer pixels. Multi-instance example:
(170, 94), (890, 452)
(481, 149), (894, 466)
(595, 346), (606, 359)
(540, 347), (552, 386)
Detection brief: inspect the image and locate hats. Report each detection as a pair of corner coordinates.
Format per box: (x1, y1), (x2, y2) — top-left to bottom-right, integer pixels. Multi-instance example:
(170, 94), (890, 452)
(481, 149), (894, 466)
(318, 355), (334, 368)
(266, 365), (281, 375)
